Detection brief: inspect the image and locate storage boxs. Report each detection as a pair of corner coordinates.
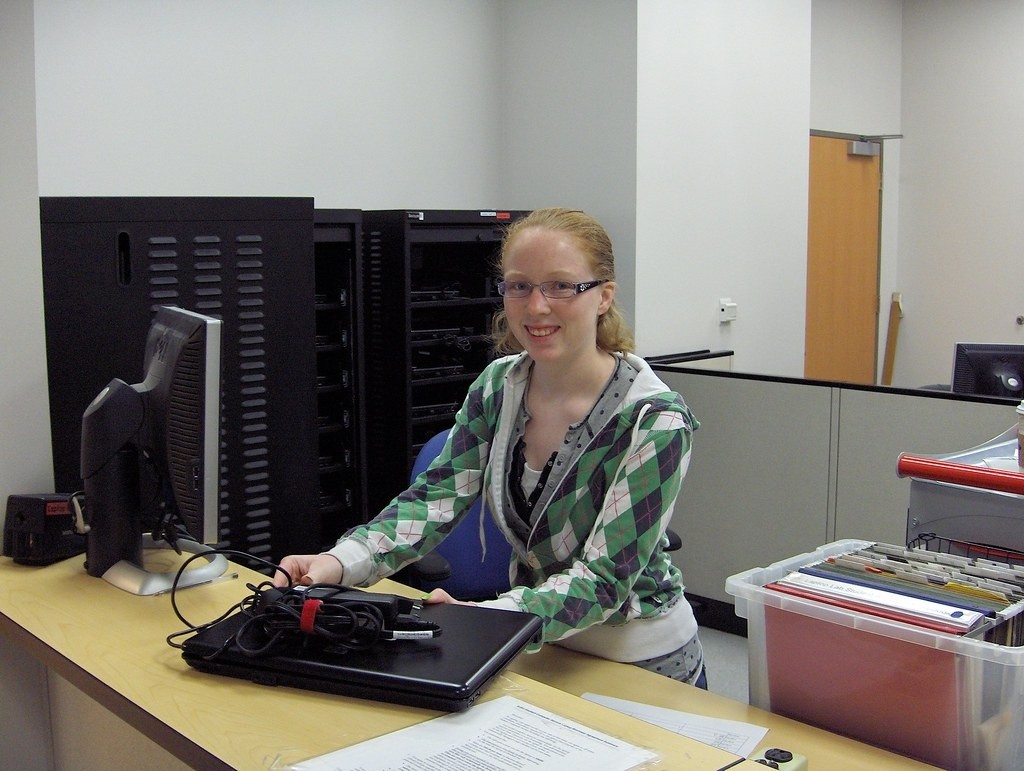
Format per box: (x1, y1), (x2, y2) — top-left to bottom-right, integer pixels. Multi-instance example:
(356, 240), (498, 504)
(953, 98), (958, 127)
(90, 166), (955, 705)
(724, 538), (1023, 771)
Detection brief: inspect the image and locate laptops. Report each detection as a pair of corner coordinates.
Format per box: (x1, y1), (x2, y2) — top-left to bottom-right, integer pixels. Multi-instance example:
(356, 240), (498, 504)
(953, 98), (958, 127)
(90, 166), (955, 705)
(183, 588), (543, 711)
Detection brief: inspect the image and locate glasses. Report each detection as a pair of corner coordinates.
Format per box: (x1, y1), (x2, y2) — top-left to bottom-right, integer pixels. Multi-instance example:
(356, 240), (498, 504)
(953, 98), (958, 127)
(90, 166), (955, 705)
(496, 278), (609, 299)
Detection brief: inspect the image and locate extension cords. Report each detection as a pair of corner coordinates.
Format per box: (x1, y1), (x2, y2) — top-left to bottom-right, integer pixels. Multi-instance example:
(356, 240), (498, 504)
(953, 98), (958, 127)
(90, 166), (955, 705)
(751, 746), (809, 771)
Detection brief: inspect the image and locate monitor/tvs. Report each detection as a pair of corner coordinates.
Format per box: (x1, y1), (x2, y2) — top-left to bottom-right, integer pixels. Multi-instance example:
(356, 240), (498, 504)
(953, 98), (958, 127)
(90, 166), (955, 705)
(78, 305), (227, 600)
(950, 342), (1024, 400)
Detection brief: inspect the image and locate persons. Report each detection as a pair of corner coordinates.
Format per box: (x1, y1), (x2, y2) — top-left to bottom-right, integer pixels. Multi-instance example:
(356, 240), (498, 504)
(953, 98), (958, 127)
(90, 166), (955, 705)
(273, 209), (709, 689)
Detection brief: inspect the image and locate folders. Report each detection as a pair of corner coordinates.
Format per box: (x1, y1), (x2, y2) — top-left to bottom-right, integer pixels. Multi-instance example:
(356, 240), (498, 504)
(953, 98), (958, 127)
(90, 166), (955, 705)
(763, 567), (996, 771)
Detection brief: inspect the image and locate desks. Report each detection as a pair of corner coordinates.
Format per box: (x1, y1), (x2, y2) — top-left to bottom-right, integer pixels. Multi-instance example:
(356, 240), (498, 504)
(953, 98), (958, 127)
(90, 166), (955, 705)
(0, 521), (944, 771)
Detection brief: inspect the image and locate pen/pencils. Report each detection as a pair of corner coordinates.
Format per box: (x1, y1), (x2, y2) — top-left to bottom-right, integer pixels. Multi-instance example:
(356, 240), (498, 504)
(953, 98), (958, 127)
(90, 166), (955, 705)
(154, 571), (239, 596)
